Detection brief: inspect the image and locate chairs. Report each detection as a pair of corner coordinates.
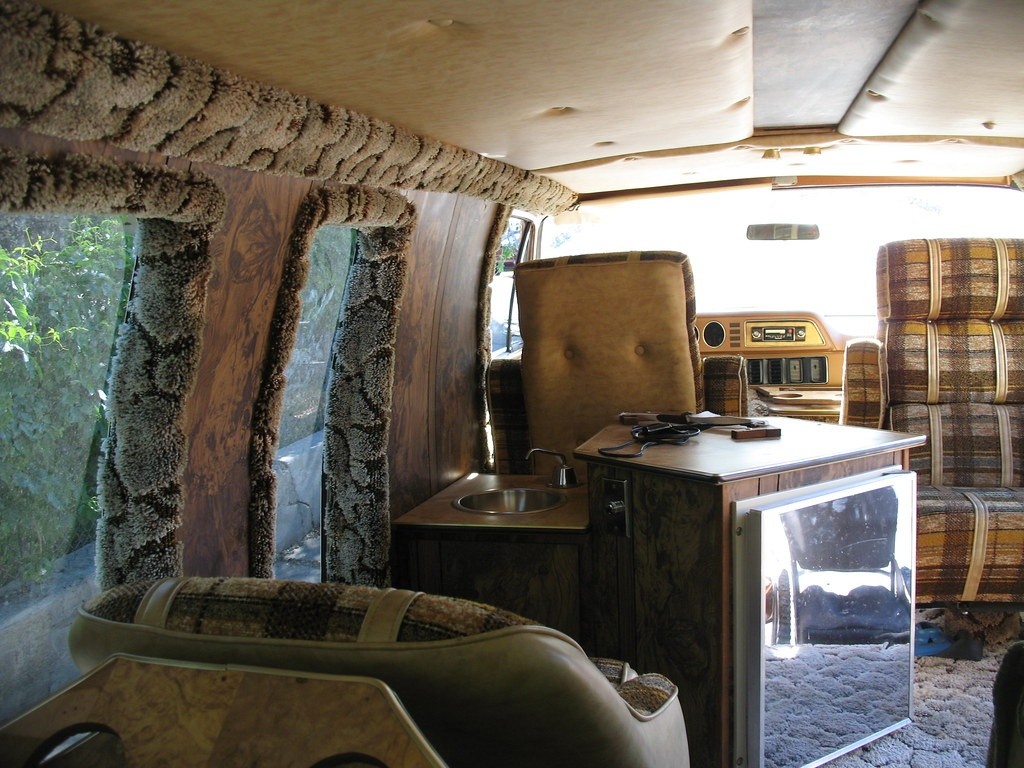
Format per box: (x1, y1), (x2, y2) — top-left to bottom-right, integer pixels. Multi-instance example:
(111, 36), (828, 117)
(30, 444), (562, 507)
(69, 577), (691, 768)
(840, 239), (1024, 608)
(485, 251), (748, 475)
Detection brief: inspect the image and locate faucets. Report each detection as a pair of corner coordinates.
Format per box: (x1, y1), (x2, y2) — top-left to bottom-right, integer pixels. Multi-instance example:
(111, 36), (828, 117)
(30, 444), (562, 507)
(525, 448), (579, 487)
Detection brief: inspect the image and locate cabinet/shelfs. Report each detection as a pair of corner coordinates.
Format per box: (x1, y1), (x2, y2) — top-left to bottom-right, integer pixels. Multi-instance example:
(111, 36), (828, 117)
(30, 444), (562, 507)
(390, 524), (592, 659)
(574, 415), (927, 768)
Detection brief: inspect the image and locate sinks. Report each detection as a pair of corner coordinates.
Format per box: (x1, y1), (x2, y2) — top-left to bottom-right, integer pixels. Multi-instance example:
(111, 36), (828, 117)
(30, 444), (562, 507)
(452, 488), (568, 513)
